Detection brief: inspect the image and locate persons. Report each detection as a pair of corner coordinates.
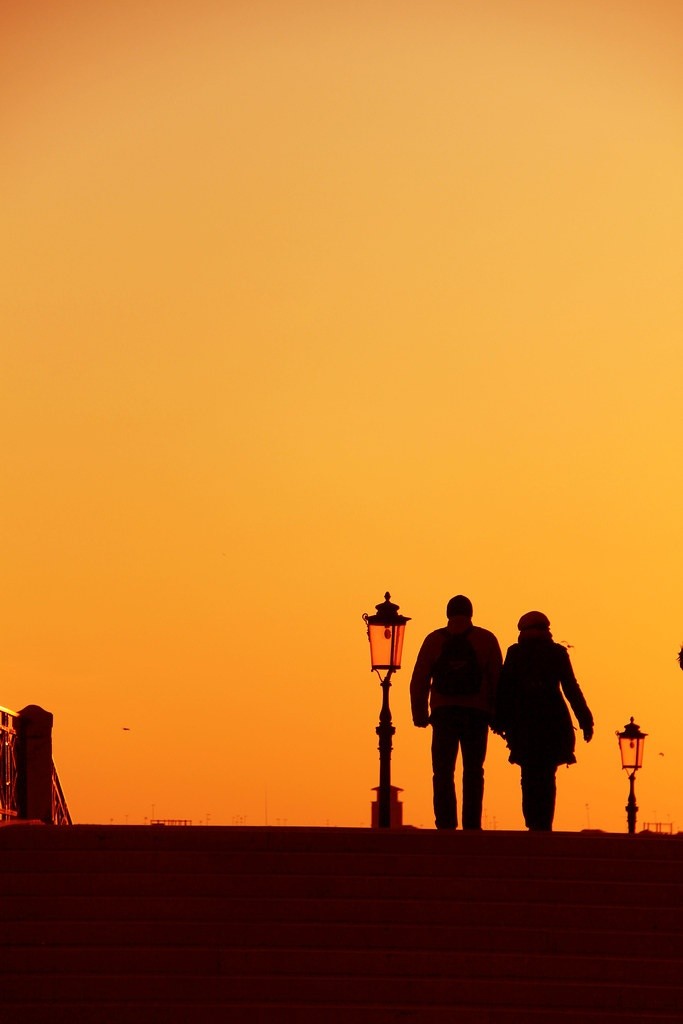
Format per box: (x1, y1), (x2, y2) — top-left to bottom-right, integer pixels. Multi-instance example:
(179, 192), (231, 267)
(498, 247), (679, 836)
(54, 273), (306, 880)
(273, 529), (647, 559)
(496, 612), (595, 831)
(411, 595), (502, 830)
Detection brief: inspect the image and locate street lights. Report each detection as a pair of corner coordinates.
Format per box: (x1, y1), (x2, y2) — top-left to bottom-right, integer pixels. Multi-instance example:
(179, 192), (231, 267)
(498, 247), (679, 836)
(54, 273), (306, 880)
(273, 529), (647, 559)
(362, 591), (412, 828)
(615, 717), (648, 834)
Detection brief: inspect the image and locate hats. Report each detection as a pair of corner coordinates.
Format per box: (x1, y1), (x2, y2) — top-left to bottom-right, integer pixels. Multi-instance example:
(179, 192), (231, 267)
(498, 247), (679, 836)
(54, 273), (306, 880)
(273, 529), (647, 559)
(517, 610), (550, 631)
(447, 595), (473, 618)
